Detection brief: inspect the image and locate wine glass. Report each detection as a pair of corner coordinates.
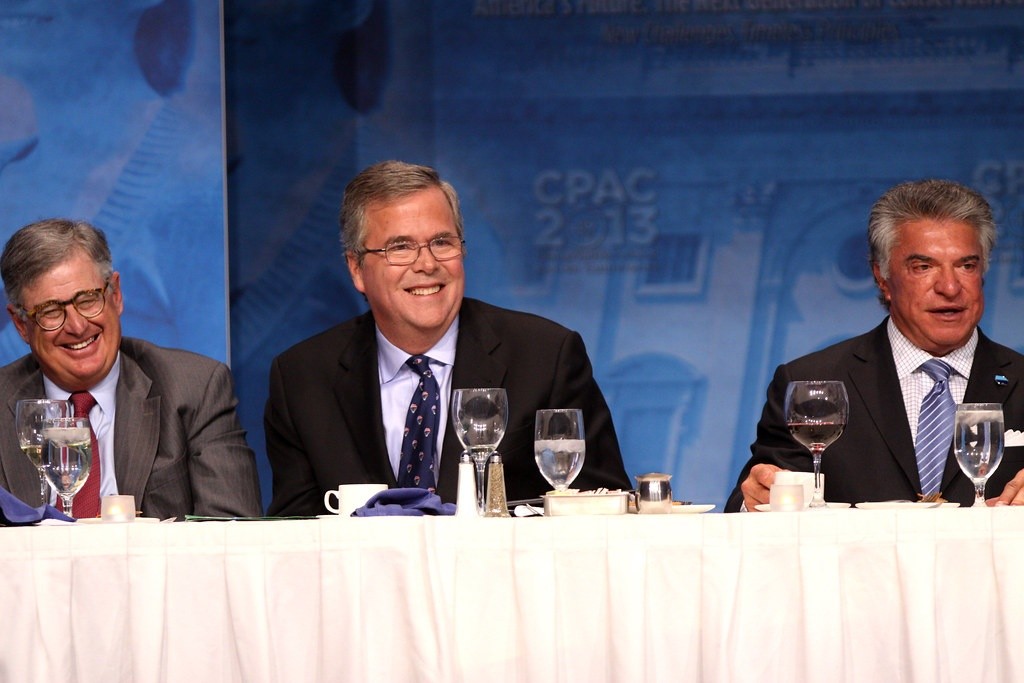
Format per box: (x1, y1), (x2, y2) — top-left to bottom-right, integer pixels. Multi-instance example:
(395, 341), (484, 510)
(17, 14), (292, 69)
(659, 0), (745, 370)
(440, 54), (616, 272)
(39, 418), (92, 521)
(953, 403), (1005, 509)
(533, 408), (586, 492)
(784, 380), (849, 511)
(16, 400), (74, 513)
(452, 387), (509, 520)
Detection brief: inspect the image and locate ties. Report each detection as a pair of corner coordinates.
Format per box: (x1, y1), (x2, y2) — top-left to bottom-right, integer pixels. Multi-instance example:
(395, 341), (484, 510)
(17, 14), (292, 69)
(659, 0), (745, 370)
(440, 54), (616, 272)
(398, 355), (441, 489)
(57, 392), (100, 517)
(914, 360), (956, 497)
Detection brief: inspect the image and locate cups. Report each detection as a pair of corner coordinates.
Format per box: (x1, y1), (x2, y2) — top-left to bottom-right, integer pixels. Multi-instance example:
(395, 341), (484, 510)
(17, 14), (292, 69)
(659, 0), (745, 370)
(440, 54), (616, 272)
(324, 484), (391, 517)
(771, 473), (825, 506)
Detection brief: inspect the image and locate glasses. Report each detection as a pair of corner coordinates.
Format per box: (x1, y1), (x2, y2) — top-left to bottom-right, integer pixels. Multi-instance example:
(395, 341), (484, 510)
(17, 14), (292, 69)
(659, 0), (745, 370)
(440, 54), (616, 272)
(16, 280), (109, 330)
(358, 239), (465, 264)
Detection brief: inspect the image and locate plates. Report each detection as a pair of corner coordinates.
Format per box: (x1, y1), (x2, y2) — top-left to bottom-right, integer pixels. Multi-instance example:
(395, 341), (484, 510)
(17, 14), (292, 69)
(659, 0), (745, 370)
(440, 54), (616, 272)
(625, 504), (715, 516)
(755, 502), (853, 512)
(856, 502), (961, 510)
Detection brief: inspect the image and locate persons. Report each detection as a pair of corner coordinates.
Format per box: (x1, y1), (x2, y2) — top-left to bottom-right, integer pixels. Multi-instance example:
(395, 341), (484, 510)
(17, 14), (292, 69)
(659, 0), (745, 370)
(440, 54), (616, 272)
(265, 160), (634, 516)
(0, 217), (259, 517)
(724, 178), (1024, 513)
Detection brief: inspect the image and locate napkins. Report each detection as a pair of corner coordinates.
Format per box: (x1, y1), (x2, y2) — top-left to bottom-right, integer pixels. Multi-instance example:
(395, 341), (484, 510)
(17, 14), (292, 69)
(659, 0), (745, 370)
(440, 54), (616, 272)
(349, 487), (457, 516)
(0, 485), (77, 525)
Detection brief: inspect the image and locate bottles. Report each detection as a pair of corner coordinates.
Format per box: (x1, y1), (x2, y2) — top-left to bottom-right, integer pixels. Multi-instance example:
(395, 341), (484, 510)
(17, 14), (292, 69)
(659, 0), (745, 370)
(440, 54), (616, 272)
(455, 451), (481, 518)
(484, 449), (508, 518)
